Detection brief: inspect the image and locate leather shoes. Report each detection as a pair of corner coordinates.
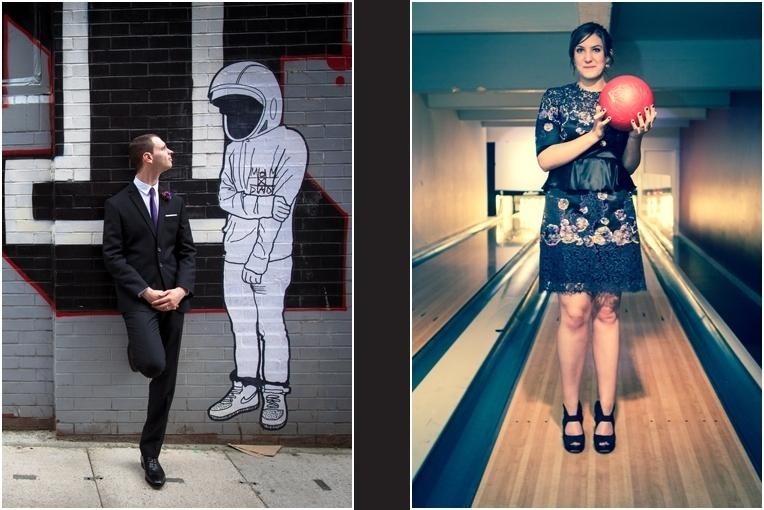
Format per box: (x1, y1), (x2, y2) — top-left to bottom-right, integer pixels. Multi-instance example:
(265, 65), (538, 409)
(141, 453), (166, 486)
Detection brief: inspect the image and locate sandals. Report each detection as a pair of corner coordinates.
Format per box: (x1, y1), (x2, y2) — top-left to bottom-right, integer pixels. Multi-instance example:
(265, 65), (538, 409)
(595, 399), (615, 454)
(563, 399), (584, 453)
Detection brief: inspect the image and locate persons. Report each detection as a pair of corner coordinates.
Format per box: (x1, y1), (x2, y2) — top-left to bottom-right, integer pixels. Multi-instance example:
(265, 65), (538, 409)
(101, 133), (198, 490)
(532, 20), (659, 455)
(206, 59), (311, 431)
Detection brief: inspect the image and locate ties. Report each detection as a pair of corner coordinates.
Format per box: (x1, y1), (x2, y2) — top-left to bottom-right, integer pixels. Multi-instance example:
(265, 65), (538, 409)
(149, 187), (158, 233)
